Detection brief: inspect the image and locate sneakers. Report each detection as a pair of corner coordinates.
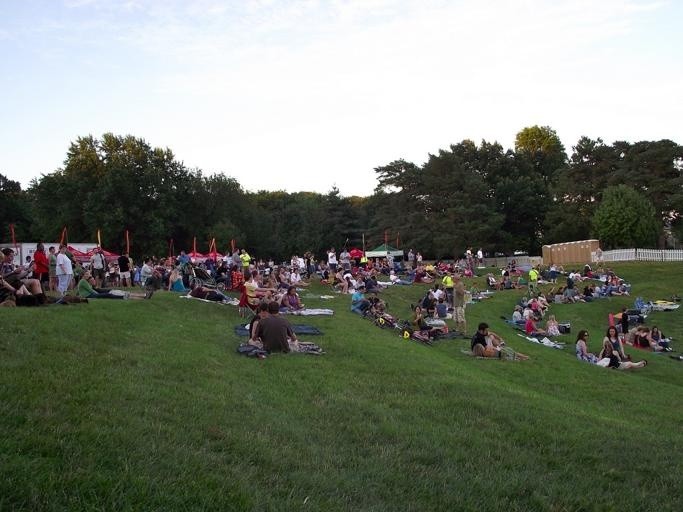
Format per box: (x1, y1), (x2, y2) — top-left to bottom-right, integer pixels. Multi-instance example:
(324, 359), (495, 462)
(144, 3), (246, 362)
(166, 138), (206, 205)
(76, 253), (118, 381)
(123, 293), (127, 300)
(144, 291), (152, 299)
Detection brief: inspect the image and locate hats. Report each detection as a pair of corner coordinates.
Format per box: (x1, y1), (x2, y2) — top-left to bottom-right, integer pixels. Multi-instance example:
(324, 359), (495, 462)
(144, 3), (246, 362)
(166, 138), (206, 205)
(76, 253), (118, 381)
(514, 305), (521, 311)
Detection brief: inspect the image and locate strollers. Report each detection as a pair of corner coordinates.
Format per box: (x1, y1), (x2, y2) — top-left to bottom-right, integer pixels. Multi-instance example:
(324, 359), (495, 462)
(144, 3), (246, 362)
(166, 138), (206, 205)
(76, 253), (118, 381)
(363, 303), (432, 345)
(188, 261), (226, 292)
(486, 272), (504, 291)
